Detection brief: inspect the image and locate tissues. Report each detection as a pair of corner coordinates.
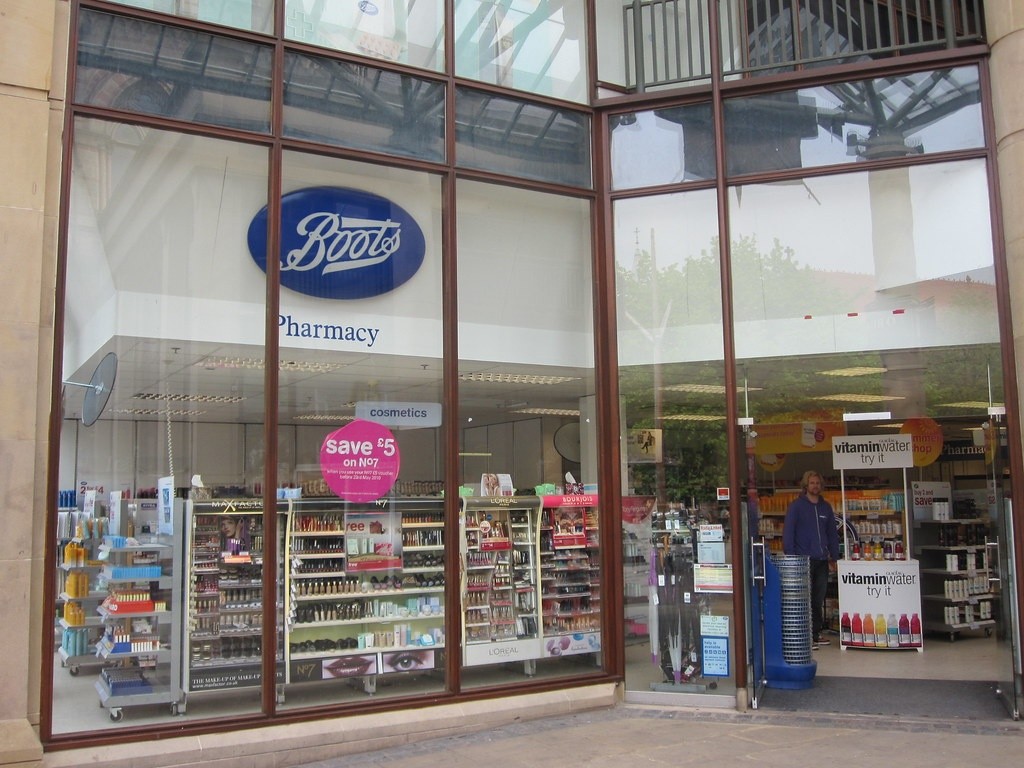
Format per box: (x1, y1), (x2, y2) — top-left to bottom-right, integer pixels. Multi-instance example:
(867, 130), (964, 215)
(565, 471), (584, 496)
(190, 474), (211, 503)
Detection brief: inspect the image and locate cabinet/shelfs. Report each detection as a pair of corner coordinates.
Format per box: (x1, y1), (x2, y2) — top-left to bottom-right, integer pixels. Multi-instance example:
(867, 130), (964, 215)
(57, 490), (129, 677)
(914, 519), (996, 642)
(94, 496), (186, 722)
(759, 490), (906, 554)
(52, 506), (79, 652)
(177, 494), (601, 717)
(621, 494), (659, 647)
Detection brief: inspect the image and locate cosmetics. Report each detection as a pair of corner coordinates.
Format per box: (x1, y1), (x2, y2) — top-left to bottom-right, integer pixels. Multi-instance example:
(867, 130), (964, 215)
(190, 509), (600, 660)
(545, 639), (562, 656)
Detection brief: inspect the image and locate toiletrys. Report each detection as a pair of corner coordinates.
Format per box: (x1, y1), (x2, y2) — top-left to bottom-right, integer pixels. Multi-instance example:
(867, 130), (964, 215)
(932, 497), (992, 625)
(57, 489), (108, 658)
(759, 491), (905, 633)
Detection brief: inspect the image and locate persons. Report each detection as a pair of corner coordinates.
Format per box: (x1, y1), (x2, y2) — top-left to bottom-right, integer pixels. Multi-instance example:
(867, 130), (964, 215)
(221, 516), (245, 554)
(482, 474), (501, 496)
(322, 656), (376, 680)
(384, 650), (435, 673)
(784, 471), (841, 650)
(642, 431), (655, 453)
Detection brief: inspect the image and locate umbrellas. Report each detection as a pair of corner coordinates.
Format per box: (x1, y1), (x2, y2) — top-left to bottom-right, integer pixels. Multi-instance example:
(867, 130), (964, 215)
(649, 535), (703, 685)
